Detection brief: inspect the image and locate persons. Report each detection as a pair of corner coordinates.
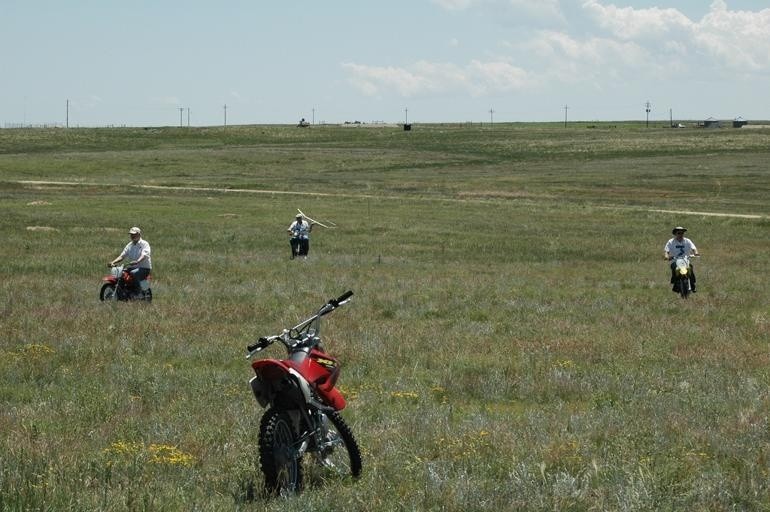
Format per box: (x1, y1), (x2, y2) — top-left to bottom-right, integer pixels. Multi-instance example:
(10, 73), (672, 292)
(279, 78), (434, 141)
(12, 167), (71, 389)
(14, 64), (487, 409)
(664, 226), (699, 293)
(107, 227), (152, 300)
(287, 214), (315, 259)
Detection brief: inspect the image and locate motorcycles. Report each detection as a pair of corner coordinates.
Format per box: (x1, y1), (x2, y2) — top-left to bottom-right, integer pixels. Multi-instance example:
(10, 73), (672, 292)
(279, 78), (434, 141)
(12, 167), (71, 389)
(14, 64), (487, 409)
(245, 289), (364, 498)
(664, 254), (701, 298)
(99, 261), (152, 305)
(287, 227), (313, 260)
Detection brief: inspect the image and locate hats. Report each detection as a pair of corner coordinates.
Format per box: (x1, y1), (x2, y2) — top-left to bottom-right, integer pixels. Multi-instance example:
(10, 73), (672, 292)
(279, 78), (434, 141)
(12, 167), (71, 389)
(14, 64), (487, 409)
(128, 227), (141, 235)
(296, 213), (302, 218)
(673, 226), (687, 235)
